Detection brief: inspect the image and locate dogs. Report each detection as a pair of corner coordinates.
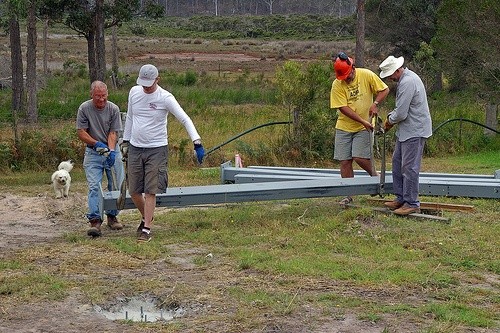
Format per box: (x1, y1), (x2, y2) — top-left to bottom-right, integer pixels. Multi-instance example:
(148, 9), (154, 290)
(51, 159), (74, 199)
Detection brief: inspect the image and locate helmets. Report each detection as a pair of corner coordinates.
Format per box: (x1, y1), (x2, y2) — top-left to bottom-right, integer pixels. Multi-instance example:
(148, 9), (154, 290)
(334, 57), (353, 80)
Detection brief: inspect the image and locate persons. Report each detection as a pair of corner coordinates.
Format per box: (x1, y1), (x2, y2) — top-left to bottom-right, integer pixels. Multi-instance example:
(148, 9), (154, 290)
(122, 64), (204, 242)
(379, 55), (432, 215)
(76, 81), (123, 236)
(329, 54), (390, 205)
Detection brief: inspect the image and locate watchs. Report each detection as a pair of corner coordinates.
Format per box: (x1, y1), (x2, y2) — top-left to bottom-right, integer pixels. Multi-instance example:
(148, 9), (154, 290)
(374, 102), (379, 106)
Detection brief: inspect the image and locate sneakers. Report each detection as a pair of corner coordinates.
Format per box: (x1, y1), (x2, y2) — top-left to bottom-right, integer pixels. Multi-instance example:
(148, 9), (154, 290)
(138, 227), (151, 242)
(137, 217), (154, 232)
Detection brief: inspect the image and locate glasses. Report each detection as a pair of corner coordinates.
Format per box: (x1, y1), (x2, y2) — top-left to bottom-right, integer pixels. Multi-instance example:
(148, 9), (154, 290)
(92, 94), (107, 99)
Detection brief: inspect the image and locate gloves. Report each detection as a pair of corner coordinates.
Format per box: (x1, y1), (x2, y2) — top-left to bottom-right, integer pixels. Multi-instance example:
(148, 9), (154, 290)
(106, 151), (115, 167)
(194, 144), (204, 164)
(384, 114), (394, 131)
(94, 141), (107, 152)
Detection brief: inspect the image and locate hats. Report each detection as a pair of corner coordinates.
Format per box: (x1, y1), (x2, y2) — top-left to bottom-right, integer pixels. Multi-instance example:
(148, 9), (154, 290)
(136, 64), (158, 87)
(379, 56), (404, 78)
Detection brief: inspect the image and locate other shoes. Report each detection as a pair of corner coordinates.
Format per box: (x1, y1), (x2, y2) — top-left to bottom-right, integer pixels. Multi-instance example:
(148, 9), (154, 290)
(87, 219), (101, 236)
(394, 205), (420, 215)
(384, 201), (402, 207)
(342, 195), (354, 203)
(108, 216), (123, 229)
